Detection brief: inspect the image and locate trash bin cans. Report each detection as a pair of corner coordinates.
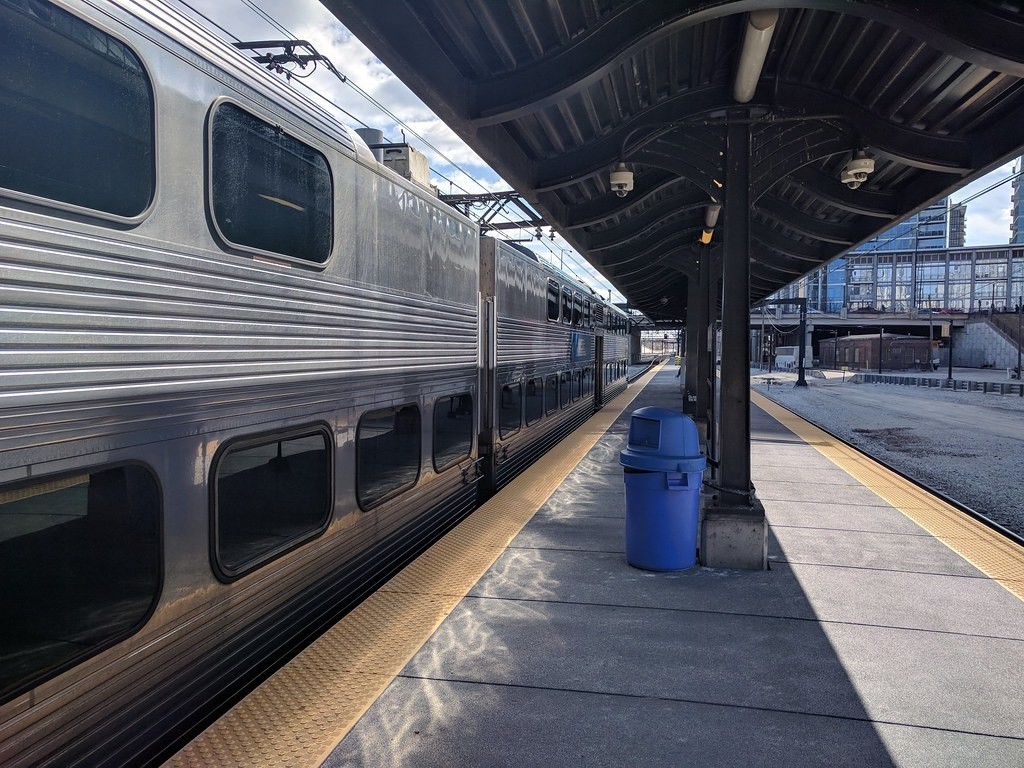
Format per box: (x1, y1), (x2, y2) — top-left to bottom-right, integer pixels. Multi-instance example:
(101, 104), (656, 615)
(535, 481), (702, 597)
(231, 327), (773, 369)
(617, 406), (709, 573)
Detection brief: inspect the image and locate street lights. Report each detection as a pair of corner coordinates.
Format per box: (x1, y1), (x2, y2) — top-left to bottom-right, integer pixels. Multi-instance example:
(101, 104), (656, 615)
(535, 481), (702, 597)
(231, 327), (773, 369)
(809, 296), (812, 316)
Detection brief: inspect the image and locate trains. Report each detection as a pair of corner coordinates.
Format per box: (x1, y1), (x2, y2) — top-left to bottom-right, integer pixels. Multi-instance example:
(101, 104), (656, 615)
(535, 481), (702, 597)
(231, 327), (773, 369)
(0, 0), (629, 768)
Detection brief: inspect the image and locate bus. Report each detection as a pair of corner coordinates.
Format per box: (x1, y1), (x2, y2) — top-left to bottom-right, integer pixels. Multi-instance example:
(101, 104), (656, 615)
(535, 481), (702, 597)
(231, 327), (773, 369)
(771, 344), (814, 370)
